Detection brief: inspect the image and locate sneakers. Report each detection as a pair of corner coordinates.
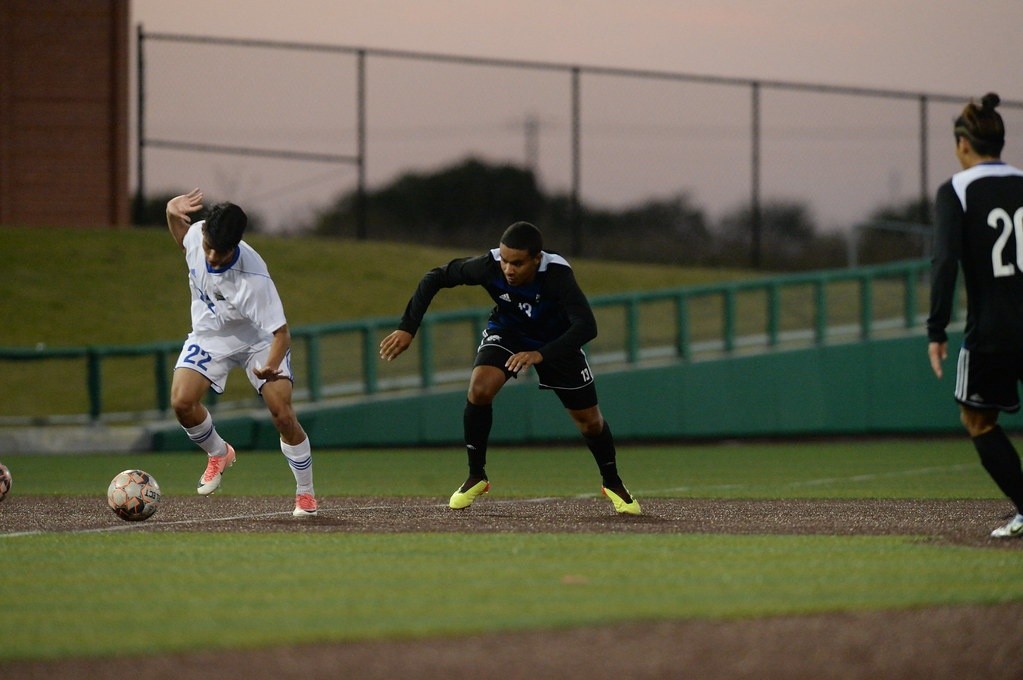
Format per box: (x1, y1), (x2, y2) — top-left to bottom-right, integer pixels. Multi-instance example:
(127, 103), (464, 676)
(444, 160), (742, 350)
(449, 472), (490, 509)
(197, 441), (236, 498)
(293, 491), (317, 517)
(990, 513), (1023, 539)
(601, 477), (641, 515)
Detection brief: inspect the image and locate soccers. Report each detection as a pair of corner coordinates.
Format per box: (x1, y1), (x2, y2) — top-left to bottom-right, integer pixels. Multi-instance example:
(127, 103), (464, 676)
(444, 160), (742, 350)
(107, 468), (161, 524)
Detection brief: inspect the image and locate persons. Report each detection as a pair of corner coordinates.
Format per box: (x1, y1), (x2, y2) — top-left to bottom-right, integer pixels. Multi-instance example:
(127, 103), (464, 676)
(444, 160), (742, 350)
(165, 187), (318, 517)
(379, 222), (643, 515)
(924, 93), (1023, 539)
